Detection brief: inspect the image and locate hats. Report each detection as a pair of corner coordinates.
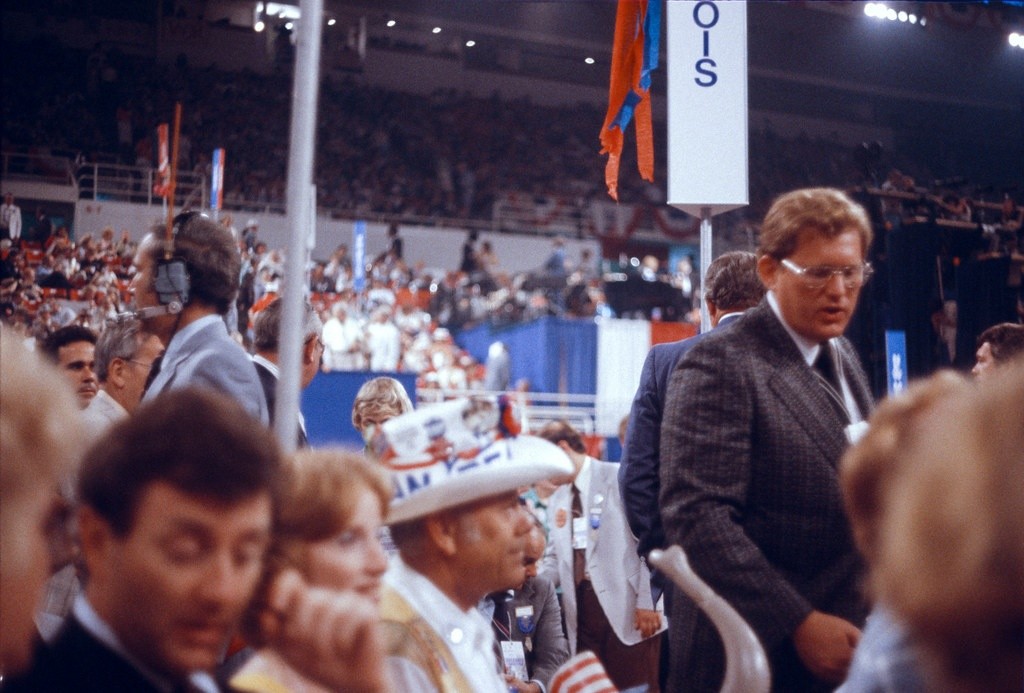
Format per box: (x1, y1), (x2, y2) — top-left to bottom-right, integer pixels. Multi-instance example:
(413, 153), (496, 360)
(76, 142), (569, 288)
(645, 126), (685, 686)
(378, 396), (576, 524)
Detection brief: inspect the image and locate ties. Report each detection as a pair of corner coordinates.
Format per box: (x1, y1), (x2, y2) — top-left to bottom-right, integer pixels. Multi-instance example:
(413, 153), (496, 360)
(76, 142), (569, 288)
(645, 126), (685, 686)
(814, 342), (847, 410)
(490, 593), (510, 645)
(569, 484), (585, 587)
(138, 350), (167, 404)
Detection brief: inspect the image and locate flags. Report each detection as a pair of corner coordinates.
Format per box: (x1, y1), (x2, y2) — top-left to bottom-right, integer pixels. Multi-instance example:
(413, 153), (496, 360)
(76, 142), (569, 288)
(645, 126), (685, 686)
(600, 0), (659, 202)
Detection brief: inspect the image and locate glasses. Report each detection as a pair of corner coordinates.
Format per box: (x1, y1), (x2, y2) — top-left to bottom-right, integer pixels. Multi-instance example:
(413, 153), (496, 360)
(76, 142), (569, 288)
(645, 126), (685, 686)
(777, 258), (873, 288)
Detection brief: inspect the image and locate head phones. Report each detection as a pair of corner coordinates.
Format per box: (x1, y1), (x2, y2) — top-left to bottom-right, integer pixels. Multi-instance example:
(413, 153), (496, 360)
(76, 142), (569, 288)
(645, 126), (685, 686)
(154, 210), (209, 308)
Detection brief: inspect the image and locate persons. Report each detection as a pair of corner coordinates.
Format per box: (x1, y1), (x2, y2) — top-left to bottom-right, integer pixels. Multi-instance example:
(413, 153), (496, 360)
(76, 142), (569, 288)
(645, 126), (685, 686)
(250, 296), (325, 449)
(0, 190), (287, 436)
(827, 320), (1024, 693)
(226, 447), (445, 693)
(616, 250), (770, 612)
(306, 220), (701, 449)
(0, 384), (288, 693)
(0, 326), (88, 674)
(350, 378), (666, 693)
(660, 185), (879, 693)
(0, 40), (1024, 280)
(883, 168), (1024, 354)
(129, 212), (270, 432)
